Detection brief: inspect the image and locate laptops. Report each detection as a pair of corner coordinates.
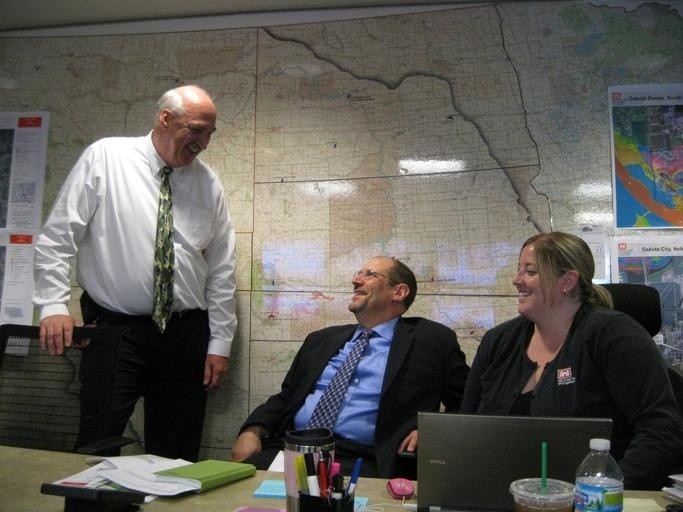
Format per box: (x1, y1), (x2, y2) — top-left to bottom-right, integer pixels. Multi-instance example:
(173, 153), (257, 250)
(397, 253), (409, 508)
(417, 413), (613, 512)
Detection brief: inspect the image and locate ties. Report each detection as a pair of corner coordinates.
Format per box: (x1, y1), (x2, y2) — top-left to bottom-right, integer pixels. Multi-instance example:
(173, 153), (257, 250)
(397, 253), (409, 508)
(307, 329), (374, 432)
(152, 166), (174, 332)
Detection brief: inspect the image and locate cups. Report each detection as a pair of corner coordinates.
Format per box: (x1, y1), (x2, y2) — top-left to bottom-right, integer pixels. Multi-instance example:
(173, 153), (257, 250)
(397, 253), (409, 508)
(283, 426), (336, 512)
(298, 488), (355, 512)
(507, 476), (576, 512)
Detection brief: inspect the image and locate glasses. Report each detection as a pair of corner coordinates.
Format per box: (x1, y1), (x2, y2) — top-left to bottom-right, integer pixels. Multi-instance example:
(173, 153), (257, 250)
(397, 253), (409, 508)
(354, 270), (401, 284)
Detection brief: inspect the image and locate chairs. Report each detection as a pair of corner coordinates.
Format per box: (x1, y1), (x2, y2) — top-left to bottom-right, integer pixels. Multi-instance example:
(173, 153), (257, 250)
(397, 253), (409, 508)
(603, 285), (683, 404)
(2, 323), (134, 457)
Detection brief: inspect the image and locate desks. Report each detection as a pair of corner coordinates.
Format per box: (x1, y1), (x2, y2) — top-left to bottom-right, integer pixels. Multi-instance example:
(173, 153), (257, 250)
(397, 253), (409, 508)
(0, 444), (683, 511)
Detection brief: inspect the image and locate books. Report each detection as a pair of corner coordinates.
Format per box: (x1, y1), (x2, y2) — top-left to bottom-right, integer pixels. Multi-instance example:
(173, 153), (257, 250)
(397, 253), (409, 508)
(151, 458), (257, 497)
(666, 473), (682, 491)
(659, 486), (682, 505)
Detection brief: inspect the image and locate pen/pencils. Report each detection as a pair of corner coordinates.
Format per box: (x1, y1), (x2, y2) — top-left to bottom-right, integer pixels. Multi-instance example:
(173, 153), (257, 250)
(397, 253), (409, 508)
(294, 451), (363, 500)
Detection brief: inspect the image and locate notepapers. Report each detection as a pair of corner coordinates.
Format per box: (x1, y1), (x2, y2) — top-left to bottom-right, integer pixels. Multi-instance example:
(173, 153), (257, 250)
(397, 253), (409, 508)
(253, 479), (287, 498)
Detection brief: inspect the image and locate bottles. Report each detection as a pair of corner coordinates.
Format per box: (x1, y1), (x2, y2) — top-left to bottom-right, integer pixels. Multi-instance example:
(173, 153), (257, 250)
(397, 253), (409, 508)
(573, 437), (625, 512)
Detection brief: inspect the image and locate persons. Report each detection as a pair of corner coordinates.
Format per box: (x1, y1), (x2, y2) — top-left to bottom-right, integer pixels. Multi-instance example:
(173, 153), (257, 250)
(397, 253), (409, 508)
(456, 228), (682, 491)
(30, 83), (239, 465)
(228, 253), (472, 476)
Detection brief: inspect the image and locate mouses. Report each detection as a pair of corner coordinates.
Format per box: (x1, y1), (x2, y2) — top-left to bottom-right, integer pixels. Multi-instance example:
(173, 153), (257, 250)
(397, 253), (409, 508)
(386, 479), (414, 500)
